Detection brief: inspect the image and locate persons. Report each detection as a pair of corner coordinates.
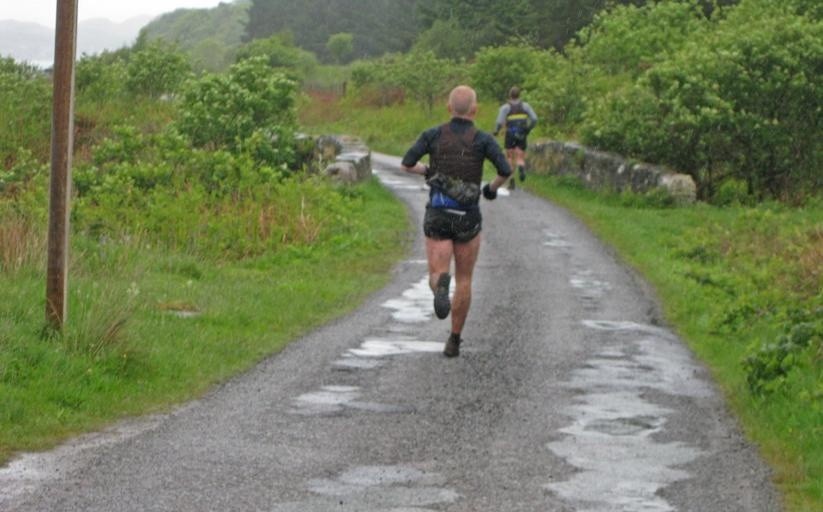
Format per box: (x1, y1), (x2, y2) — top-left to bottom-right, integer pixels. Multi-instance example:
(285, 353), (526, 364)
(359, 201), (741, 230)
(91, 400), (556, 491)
(400, 84), (511, 358)
(492, 85), (538, 192)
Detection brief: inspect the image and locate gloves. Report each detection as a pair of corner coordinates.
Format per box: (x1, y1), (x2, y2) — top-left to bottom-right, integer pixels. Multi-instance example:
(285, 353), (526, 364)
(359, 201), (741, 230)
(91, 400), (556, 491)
(483, 183), (497, 200)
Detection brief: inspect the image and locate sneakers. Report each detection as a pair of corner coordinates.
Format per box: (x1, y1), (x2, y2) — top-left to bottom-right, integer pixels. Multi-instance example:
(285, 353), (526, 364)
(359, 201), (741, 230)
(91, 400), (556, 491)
(508, 182), (515, 189)
(434, 272), (452, 320)
(443, 340), (461, 356)
(518, 165), (526, 182)
